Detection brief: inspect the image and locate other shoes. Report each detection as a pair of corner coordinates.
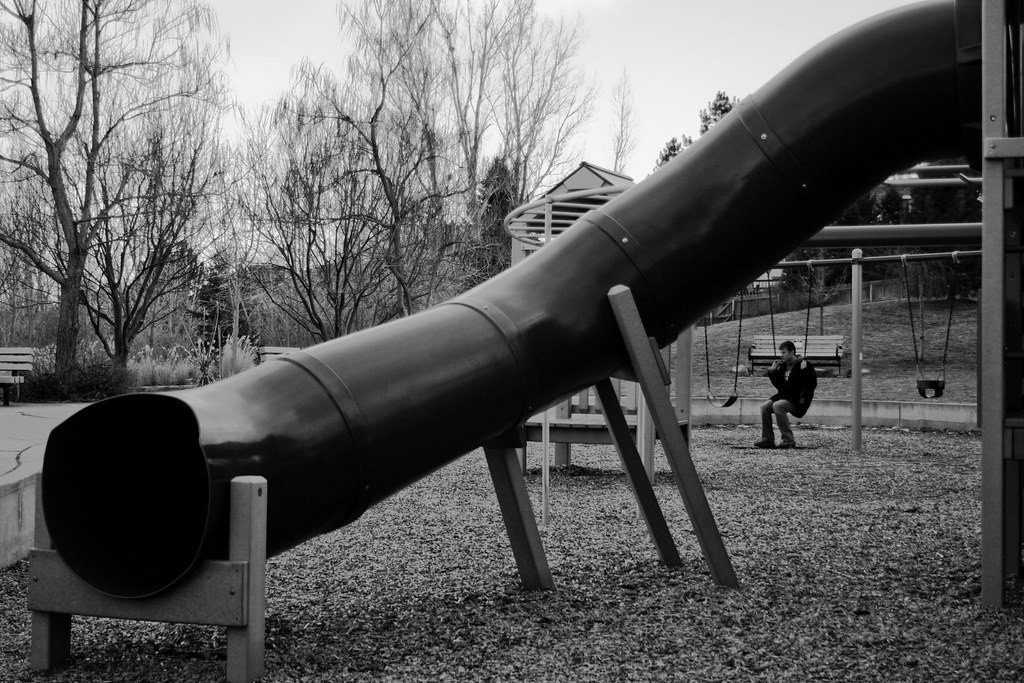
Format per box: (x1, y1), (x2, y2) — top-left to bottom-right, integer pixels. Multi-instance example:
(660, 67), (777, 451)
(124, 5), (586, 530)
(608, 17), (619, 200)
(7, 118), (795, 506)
(775, 440), (795, 448)
(754, 440), (775, 449)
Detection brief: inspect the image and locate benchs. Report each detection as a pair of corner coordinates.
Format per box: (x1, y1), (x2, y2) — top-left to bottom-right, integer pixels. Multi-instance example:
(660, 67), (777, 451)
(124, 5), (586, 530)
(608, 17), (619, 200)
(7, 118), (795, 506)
(747, 335), (845, 376)
(0, 347), (35, 407)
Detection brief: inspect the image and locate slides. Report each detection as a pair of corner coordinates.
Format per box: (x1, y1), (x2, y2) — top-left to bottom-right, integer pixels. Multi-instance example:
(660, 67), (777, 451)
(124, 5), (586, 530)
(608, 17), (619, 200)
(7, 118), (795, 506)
(33, 1), (1023, 603)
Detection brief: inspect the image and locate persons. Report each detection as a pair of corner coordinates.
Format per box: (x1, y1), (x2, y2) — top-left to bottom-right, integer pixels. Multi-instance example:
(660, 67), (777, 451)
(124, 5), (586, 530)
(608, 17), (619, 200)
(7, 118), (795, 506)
(754, 341), (817, 449)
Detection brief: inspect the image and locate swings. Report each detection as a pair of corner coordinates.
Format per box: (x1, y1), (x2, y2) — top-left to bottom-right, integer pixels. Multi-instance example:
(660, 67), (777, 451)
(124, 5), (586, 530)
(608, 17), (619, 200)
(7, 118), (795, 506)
(764, 265), (814, 413)
(703, 292), (744, 407)
(902, 261), (960, 400)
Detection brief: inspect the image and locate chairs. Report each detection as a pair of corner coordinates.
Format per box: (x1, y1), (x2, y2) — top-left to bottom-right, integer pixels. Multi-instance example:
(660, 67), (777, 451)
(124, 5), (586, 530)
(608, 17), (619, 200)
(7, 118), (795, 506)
(260, 346), (301, 366)
